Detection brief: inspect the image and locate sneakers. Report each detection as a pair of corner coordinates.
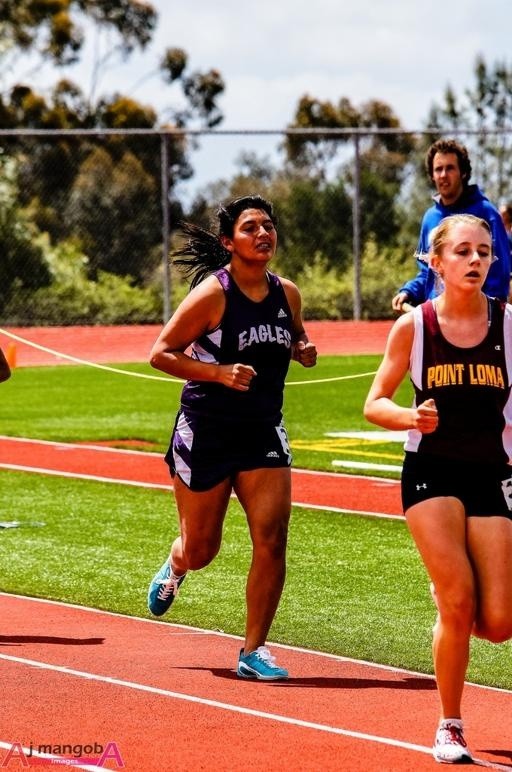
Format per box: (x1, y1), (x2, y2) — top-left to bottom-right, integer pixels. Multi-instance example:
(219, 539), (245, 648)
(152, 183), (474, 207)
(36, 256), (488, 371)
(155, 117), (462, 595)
(147, 553), (188, 617)
(237, 647), (290, 682)
(429, 715), (474, 765)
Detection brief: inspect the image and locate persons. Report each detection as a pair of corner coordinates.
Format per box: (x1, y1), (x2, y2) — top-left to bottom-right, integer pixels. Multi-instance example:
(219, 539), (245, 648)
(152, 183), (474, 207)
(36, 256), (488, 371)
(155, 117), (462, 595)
(390, 137), (512, 312)
(143, 192), (320, 685)
(362, 213), (512, 766)
(498, 201), (512, 306)
(0, 347), (14, 386)
